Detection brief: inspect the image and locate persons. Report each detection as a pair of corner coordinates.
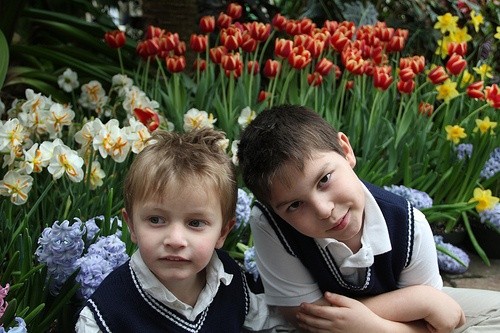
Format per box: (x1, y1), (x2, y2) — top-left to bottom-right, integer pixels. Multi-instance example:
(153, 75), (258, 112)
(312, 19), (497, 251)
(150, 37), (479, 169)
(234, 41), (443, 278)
(72, 122), (300, 332)
(236, 100), (499, 332)
